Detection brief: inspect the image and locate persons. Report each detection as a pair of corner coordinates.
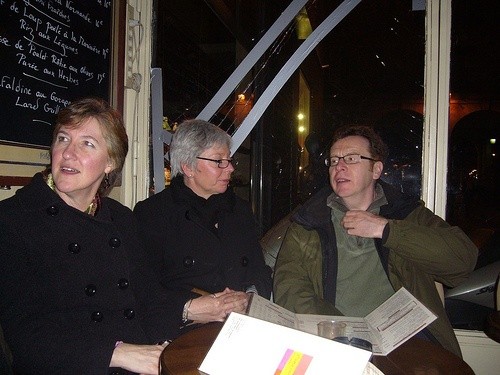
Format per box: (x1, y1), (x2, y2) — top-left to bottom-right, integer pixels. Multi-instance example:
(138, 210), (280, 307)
(272, 124), (479, 358)
(133, 120), (272, 321)
(0, 100), (180, 375)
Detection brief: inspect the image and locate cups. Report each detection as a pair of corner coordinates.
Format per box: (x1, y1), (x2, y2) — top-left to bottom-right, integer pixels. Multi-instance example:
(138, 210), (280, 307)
(317, 321), (347, 342)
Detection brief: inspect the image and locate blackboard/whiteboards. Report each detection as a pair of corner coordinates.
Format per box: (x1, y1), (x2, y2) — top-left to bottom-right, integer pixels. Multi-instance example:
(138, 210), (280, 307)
(0, 0), (114, 147)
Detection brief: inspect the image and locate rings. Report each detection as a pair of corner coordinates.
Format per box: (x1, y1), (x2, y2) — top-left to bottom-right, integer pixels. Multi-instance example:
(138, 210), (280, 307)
(213, 293), (216, 298)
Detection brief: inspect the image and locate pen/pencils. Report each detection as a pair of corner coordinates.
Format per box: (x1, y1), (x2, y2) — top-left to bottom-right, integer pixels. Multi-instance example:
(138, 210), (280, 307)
(189, 288), (215, 297)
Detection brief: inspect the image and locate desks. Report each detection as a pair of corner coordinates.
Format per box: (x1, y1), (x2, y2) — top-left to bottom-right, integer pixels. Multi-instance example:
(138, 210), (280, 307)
(158, 321), (475, 375)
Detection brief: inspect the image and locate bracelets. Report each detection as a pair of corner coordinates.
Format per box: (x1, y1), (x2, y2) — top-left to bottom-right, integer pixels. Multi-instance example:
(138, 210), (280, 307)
(183, 298), (191, 323)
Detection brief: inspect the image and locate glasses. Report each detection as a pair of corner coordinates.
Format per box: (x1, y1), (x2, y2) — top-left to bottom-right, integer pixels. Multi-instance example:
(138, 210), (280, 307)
(193, 155), (240, 170)
(322, 154), (376, 166)
